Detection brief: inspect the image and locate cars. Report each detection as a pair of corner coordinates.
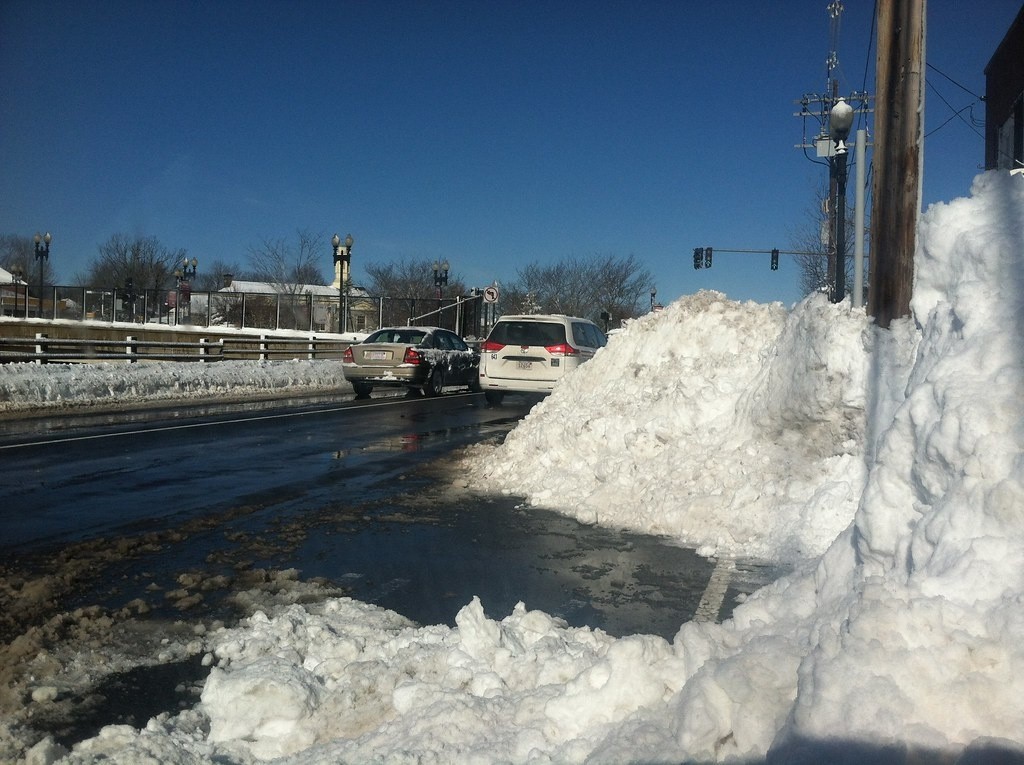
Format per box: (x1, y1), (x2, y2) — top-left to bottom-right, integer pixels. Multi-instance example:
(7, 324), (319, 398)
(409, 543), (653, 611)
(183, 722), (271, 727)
(342, 327), (480, 396)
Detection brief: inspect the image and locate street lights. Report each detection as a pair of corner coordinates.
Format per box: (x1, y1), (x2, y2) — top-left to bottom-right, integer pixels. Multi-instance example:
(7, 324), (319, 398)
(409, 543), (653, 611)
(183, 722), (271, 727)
(181, 257), (196, 283)
(34, 230), (51, 317)
(332, 234), (354, 336)
(432, 260), (450, 328)
(601, 311), (613, 335)
(651, 284), (656, 312)
(11, 264), (23, 317)
(830, 96), (855, 307)
(174, 270), (185, 329)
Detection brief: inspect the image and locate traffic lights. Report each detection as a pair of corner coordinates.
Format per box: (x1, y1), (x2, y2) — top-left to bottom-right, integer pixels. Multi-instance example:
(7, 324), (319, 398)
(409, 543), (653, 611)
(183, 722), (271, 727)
(706, 247), (713, 269)
(772, 248), (779, 270)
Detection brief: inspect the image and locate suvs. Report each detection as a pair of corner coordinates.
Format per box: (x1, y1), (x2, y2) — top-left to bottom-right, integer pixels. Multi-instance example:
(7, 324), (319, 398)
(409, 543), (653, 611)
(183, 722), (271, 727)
(479, 314), (609, 404)
